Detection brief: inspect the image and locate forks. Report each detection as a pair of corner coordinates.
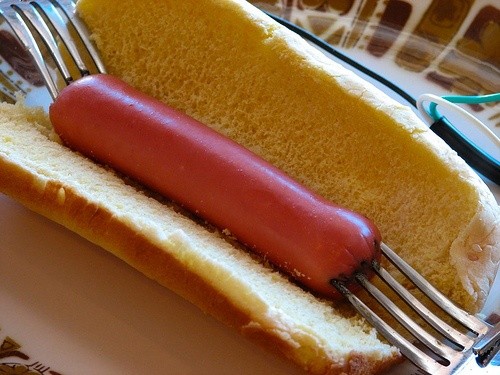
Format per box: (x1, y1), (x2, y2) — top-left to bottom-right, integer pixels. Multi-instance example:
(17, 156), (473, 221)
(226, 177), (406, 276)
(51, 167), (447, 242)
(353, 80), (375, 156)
(331, 242), (500, 374)
(0, 0), (106, 102)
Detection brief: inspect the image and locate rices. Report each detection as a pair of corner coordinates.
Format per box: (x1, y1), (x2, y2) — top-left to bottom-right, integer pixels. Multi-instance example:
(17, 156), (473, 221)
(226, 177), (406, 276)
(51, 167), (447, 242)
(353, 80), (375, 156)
(0, 1), (499, 371)
